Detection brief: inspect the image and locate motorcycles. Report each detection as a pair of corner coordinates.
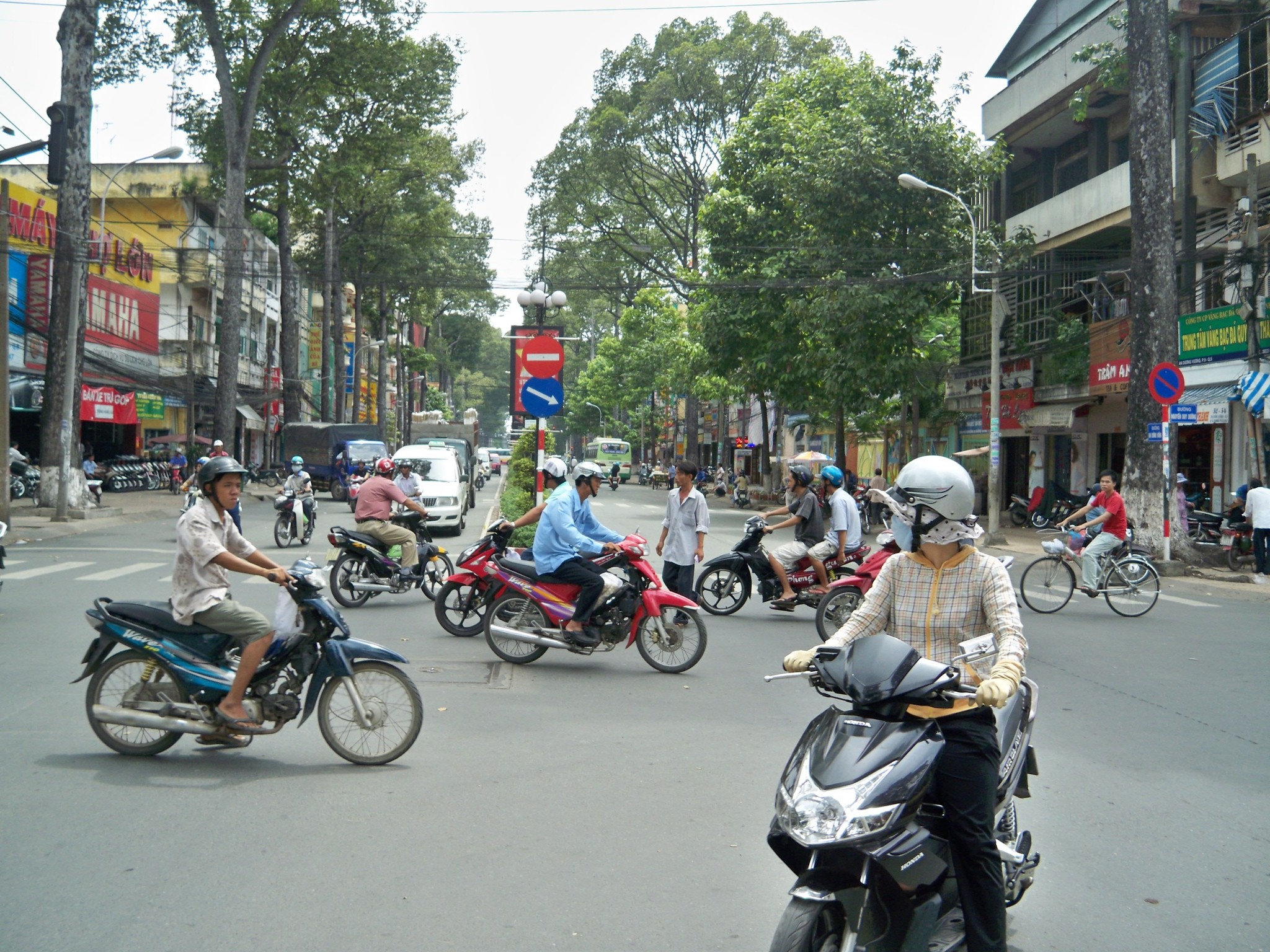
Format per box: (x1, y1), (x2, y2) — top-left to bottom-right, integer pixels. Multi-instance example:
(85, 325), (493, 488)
(68, 555), (424, 766)
(606, 468), (873, 536)
(1006, 480), (1090, 531)
(95, 455), (185, 495)
(1185, 483), (1267, 573)
(694, 515), (872, 620)
(1082, 516), (1151, 584)
(10, 451), (43, 503)
(242, 459), (286, 488)
(762, 633), (1040, 952)
(327, 493), (454, 608)
(274, 476), (318, 549)
(433, 515), (708, 673)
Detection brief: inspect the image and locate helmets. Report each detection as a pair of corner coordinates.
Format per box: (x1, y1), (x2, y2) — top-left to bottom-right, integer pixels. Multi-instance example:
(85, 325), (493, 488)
(175, 448), (182, 454)
(196, 440), (413, 496)
(571, 462), (607, 480)
(613, 460), (645, 467)
(537, 458), (567, 477)
(789, 455), (975, 520)
(739, 469), (745, 475)
(1091, 484), (1101, 495)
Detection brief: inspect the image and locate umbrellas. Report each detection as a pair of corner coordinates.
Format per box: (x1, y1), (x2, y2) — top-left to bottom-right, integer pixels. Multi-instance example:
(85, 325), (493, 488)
(147, 432), (213, 448)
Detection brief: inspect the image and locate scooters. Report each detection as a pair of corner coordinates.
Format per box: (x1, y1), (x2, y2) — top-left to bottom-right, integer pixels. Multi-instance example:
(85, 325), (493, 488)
(32, 479), (102, 506)
(815, 511), (1022, 642)
(344, 474), (365, 514)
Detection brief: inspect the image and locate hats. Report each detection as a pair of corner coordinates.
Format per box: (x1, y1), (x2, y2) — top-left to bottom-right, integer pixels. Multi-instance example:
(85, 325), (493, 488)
(865, 487), (985, 545)
(814, 474), (825, 479)
(1176, 473), (1188, 483)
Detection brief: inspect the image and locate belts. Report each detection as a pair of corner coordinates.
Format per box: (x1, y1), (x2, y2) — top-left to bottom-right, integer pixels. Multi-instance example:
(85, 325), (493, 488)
(355, 516), (381, 524)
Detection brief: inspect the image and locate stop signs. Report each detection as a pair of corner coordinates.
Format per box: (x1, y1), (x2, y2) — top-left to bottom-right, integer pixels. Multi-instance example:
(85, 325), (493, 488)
(521, 336), (565, 378)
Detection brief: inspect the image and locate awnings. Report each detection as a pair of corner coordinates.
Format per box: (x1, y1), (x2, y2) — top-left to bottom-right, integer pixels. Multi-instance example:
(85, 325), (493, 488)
(1017, 399), (1092, 429)
(950, 445), (989, 459)
(1175, 384), (1239, 405)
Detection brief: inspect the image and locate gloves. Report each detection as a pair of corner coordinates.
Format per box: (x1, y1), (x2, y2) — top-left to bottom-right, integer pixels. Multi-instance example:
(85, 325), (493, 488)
(969, 660), (1023, 709)
(784, 650), (814, 672)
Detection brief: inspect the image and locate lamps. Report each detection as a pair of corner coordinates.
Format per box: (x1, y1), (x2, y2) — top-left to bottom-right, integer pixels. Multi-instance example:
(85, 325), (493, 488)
(999, 380), (1014, 389)
(981, 383), (992, 391)
(1013, 376), (1038, 389)
(965, 382), (980, 392)
(1097, 92), (1110, 98)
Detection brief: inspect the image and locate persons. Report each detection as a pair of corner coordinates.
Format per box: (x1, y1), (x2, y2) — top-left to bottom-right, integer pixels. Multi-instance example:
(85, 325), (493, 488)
(782, 454), (1029, 952)
(1028, 450), (1045, 498)
(1071, 441), (1086, 496)
(7, 433), (1270, 646)
(167, 456), (294, 751)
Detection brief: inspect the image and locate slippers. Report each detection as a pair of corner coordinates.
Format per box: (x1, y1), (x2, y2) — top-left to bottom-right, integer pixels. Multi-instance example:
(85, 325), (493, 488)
(215, 707), (267, 731)
(560, 629), (601, 644)
(675, 588), (827, 628)
(1076, 585), (1097, 593)
(195, 736), (249, 748)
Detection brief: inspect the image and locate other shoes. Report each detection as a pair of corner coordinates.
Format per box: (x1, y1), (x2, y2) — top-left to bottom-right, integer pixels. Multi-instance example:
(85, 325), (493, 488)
(729, 499), (753, 508)
(305, 528), (313, 536)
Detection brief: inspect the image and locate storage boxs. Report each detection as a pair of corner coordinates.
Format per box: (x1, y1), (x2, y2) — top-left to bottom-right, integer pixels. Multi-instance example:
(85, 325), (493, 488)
(1181, 458), (1189, 467)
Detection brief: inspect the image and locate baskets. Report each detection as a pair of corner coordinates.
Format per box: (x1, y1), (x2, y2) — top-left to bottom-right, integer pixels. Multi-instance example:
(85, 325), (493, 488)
(273, 499), (295, 510)
(1036, 529), (1069, 555)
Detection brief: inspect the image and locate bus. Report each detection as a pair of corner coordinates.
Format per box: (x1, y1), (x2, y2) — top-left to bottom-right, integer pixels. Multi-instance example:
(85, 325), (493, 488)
(583, 437), (632, 484)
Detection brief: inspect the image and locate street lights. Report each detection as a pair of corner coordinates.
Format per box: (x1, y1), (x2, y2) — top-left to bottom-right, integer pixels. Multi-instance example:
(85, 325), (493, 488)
(896, 172), (1007, 547)
(52, 144), (186, 523)
(585, 402), (606, 438)
(515, 275), (569, 507)
(394, 375), (424, 455)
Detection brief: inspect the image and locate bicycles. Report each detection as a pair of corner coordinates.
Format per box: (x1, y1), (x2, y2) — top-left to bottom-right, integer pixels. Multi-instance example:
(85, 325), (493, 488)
(1019, 525), (1161, 618)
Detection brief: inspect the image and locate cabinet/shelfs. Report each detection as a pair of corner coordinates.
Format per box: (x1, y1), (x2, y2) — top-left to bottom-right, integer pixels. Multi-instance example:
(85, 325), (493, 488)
(1179, 467), (1211, 493)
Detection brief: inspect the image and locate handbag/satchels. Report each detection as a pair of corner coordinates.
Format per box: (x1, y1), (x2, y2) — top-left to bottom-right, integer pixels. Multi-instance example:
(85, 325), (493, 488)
(1066, 529), (1087, 560)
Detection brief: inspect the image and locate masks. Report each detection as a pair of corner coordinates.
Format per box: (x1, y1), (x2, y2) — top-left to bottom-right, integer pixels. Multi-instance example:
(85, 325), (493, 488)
(292, 465), (302, 472)
(890, 514), (926, 553)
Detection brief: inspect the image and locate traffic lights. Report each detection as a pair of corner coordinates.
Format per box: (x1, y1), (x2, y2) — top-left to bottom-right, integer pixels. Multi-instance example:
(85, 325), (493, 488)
(45, 103), (70, 184)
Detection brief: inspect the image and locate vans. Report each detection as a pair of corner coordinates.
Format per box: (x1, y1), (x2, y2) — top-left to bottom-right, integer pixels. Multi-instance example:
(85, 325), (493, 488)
(389, 420), (513, 537)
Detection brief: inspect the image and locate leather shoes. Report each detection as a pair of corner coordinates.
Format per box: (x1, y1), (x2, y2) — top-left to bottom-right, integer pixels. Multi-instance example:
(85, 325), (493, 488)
(399, 571), (423, 582)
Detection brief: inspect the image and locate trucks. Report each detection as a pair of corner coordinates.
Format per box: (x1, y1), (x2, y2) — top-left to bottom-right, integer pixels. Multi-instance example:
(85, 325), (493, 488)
(283, 422), (388, 501)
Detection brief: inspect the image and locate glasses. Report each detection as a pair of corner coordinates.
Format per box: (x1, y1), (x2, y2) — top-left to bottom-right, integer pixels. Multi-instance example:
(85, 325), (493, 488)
(400, 466), (409, 469)
